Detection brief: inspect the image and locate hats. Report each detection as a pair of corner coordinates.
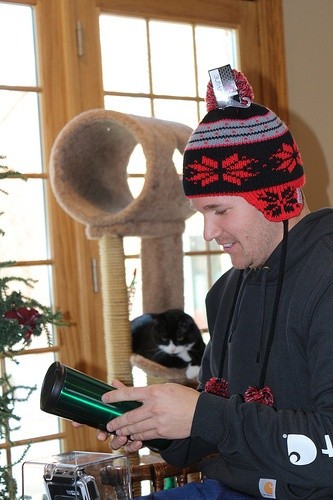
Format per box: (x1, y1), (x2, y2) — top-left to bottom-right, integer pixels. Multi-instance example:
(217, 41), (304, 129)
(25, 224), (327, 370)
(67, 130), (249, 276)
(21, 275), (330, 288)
(183, 64), (305, 407)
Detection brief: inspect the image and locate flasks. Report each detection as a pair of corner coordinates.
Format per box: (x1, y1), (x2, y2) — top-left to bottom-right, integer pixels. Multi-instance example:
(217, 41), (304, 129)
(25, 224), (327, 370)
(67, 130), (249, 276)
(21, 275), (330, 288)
(39, 362), (179, 452)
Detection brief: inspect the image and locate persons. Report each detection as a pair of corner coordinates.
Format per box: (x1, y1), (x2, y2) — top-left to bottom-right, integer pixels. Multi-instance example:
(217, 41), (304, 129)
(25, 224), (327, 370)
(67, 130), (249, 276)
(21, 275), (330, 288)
(73, 64), (333, 500)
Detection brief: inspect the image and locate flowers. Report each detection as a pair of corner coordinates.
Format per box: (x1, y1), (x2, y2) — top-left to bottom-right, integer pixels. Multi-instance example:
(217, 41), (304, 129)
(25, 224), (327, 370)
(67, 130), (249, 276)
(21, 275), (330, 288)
(0, 155), (70, 500)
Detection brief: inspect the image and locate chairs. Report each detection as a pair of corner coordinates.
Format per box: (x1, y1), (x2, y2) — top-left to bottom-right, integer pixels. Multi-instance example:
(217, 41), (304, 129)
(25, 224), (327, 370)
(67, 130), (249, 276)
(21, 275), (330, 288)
(100, 376), (219, 500)
